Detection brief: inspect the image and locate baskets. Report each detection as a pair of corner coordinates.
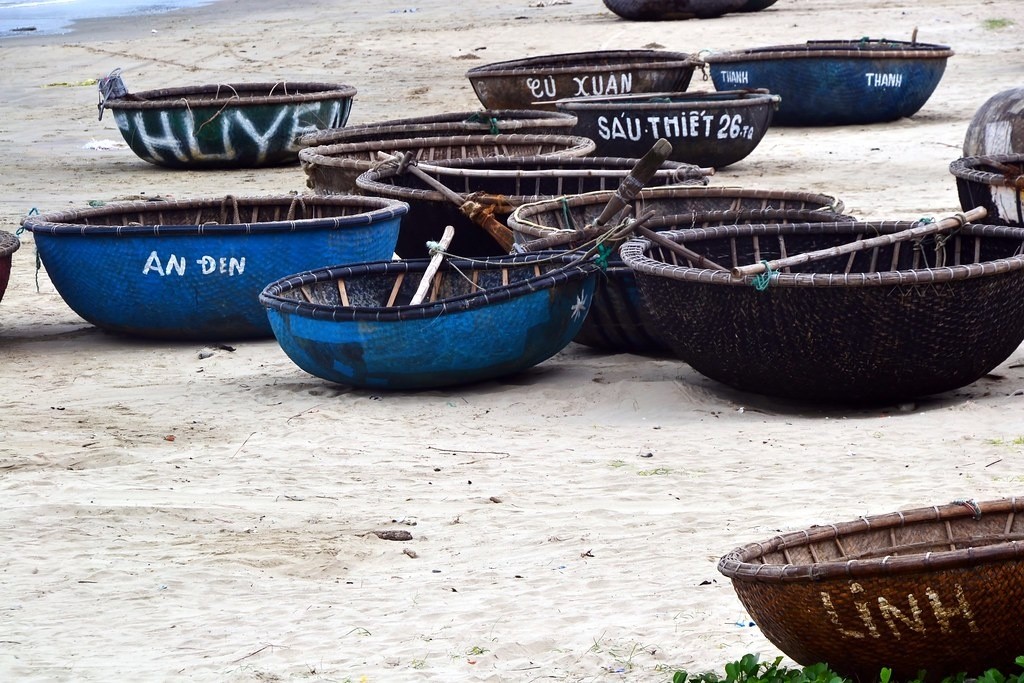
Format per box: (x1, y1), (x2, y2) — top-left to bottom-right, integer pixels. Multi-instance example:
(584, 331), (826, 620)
(21, 39), (1024, 679)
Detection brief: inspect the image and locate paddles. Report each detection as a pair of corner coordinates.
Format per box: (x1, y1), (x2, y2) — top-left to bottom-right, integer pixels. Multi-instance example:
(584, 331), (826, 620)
(393, 149), (515, 255)
